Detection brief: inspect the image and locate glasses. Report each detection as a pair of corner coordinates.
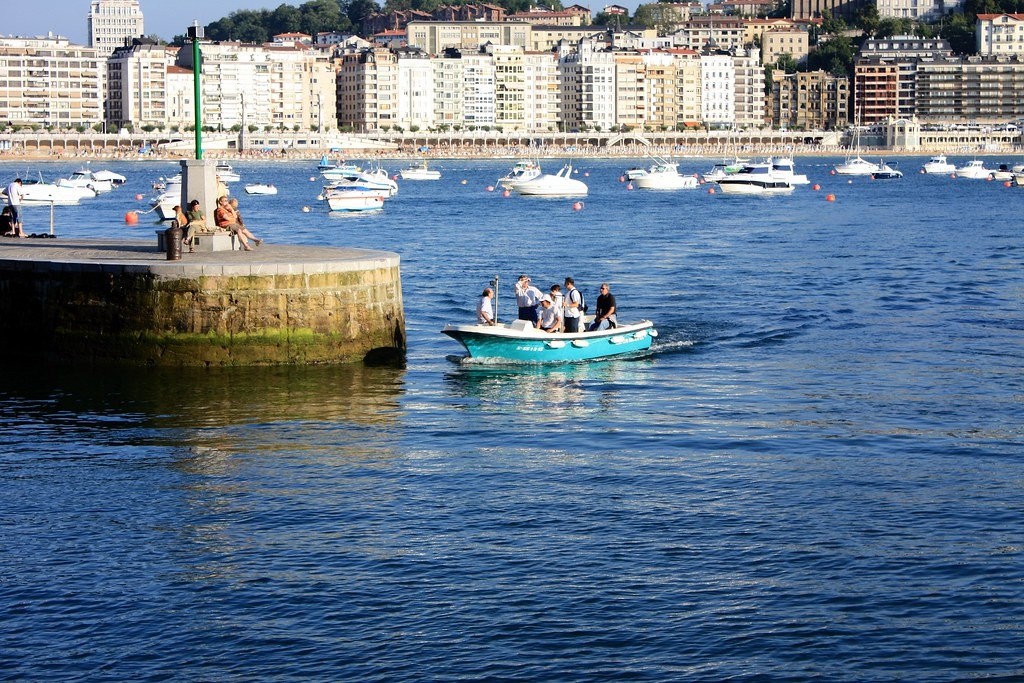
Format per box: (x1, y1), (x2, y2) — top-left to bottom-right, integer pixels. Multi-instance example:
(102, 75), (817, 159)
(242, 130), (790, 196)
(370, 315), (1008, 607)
(601, 287), (607, 290)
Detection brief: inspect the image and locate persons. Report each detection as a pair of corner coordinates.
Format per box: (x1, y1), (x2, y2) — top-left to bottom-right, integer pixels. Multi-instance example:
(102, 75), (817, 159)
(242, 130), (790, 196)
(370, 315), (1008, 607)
(0, 206), (19, 237)
(215, 175), (229, 199)
(513, 275), (544, 326)
(183, 200), (206, 252)
(535, 293), (562, 334)
(562, 276), (580, 333)
(476, 287), (494, 325)
(216, 196), (263, 251)
(2, 179), (29, 237)
(549, 285), (567, 334)
(229, 198), (244, 225)
(589, 282), (617, 331)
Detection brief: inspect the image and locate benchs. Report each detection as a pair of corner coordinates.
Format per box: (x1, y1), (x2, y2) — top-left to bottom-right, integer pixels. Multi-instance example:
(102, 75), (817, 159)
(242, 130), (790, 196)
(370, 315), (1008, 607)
(155, 229), (240, 252)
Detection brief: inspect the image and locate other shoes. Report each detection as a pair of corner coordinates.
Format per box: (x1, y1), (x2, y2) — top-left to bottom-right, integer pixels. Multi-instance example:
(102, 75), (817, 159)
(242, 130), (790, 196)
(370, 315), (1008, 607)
(245, 247), (253, 251)
(184, 240), (189, 245)
(256, 239), (263, 247)
(190, 248), (194, 252)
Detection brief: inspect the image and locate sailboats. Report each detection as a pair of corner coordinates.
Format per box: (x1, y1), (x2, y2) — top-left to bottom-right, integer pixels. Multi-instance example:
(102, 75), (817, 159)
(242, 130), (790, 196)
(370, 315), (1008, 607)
(834, 104), (881, 176)
(624, 63), (657, 181)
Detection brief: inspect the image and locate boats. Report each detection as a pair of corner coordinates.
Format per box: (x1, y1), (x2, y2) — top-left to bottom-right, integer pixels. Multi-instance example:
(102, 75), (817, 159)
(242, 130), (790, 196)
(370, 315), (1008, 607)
(634, 157), (698, 191)
(953, 158), (997, 180)
(701, 109), (813, 186)
(869, 158), (904, 178)
(990, 164), (1012, 180)
(496, 157), (544, 188)
(147, 160), (241, 220)
(508, 162), (591, 197)
(245, 181), (278, 195)
(440, 274), (659, 363)
(920, 152), (956, 174)
(714, 162), (795, 195)
(0, 170), (128, 208)
(314, 149), (400, 211)
(1011, 163), (1024, 185)
(399, 162), (444, 182)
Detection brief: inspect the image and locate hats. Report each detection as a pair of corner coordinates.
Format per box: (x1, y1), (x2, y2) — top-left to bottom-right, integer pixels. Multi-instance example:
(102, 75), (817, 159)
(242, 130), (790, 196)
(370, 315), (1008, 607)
(539, 294), (553, 303)
(191, 200), (199, 207)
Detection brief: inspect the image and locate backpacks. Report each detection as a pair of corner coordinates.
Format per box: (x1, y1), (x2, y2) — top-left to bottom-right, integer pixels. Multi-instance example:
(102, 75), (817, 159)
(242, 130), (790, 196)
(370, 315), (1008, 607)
(570, 288), (584, 311)
